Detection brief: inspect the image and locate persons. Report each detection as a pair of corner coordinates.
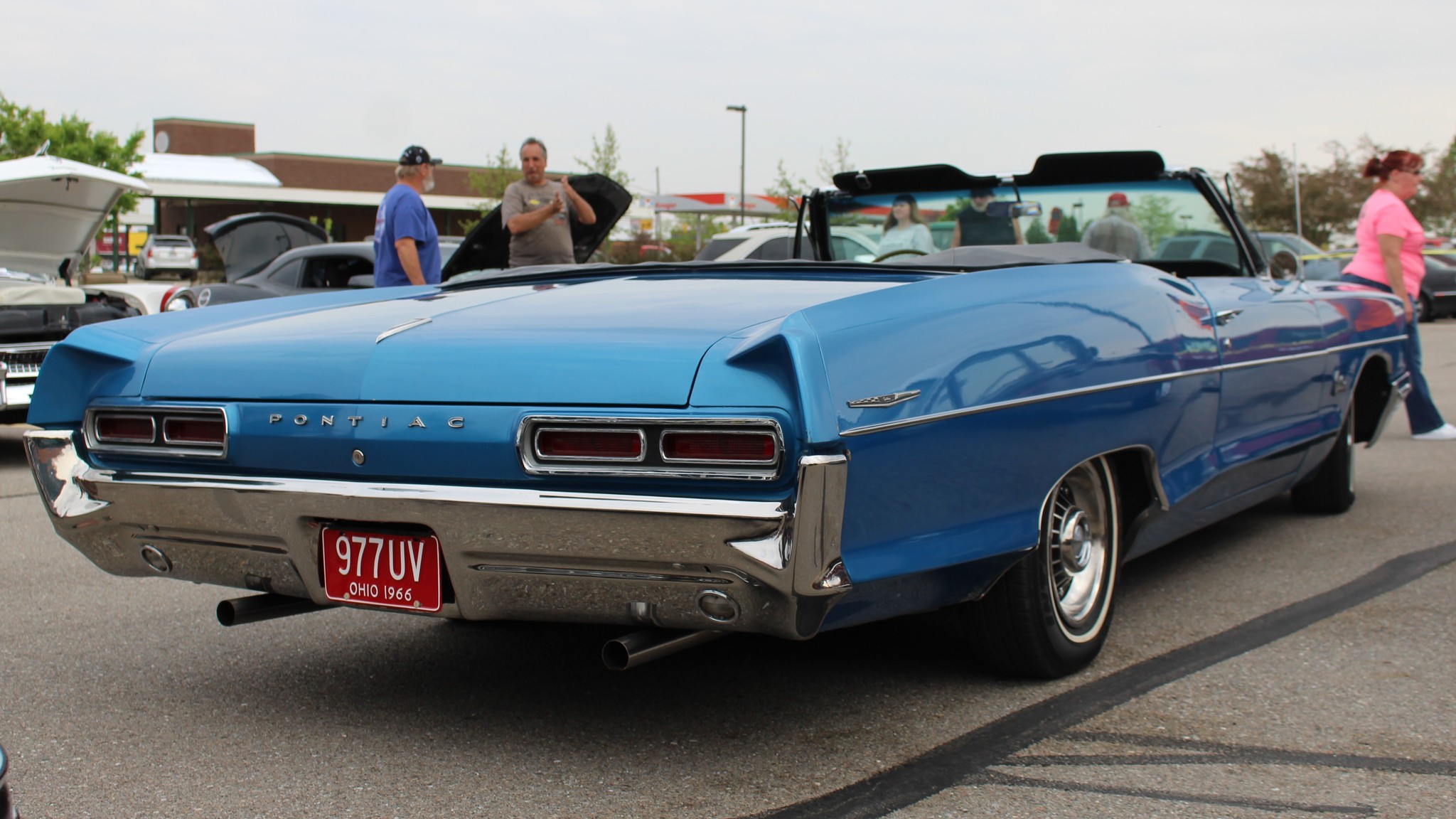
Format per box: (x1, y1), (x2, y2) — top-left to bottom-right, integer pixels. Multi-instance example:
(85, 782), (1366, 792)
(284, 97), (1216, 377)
(875, 194), (934, 262)
(1079, 192), (1154, 260)
(501, 137), (596, 268)
(373, 144), (443, 287)
(1341, 148), (1456, 440)
(951, 188), (1022, 248)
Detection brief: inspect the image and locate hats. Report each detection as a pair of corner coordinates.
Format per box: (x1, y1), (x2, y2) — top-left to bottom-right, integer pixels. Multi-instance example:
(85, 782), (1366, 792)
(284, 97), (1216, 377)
(399, 147), (444, 167)
(969, 188), (995, 197)
(1108, 193), (1130, 208)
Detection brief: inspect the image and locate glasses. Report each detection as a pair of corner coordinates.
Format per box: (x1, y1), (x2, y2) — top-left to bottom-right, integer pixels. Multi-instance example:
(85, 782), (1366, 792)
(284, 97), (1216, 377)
(1395, 167), (1420, 176)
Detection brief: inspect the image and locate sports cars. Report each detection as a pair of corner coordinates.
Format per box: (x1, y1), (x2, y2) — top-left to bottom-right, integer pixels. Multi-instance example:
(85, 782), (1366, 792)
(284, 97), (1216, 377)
(22, 149), (1413, 681)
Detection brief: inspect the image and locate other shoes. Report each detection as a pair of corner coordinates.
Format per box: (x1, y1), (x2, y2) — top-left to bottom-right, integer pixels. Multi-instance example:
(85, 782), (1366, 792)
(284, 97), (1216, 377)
(1412, 422), (1456, 440)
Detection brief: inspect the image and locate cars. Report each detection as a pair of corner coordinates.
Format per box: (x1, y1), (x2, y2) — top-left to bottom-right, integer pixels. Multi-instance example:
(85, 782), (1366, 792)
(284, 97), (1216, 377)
(133, 233), (200, 282)
(0, 153), (634, 425)
(1153, 231), (1456, 323)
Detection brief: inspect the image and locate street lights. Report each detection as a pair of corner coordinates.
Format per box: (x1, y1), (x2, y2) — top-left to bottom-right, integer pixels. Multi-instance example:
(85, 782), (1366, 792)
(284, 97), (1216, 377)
(727, 105), (747, 226)
